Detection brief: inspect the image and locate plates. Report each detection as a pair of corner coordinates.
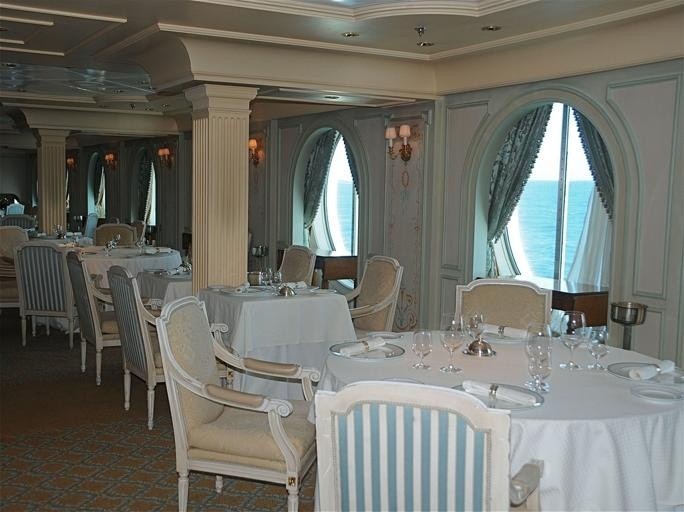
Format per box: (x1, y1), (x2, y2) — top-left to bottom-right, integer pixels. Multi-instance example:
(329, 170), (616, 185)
(328, 343), (406, 360)
(366, 331), (405, 341)
(607, 362), (682, 384)
(632, 387), (682, 403)
(450, 383), (544, 411)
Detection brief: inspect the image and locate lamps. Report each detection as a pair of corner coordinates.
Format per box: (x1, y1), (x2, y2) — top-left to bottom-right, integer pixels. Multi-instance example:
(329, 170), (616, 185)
(248, 138), (261, 166)
(385, 123), (413, 162)
(157, 147), (171, 168)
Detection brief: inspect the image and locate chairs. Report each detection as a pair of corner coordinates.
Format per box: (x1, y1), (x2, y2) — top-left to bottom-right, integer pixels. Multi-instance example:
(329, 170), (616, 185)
(456, 278), (554, 335)
(107, 265), (227, 432)
(345, 254), (403, 339)
(314, 381), (545, 511)
(155, 296), (318, 512)
(0, 200), (316, 386)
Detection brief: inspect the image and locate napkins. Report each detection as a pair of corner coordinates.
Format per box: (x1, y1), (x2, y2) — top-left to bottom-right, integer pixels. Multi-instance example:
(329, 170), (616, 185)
(630, 360), (676, 380)
(480, 324), (531, 340)
(341, 335), (386, 357)
(463, 379), (537, 407)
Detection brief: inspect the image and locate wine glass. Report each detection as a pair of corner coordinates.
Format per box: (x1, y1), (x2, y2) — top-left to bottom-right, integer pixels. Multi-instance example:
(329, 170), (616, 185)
(46, 216), (339, 298)
(413, 312), (608, 393)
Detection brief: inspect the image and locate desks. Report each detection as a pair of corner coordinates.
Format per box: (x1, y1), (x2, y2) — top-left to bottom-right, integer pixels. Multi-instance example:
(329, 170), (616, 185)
(200, 283), (359, 400)
(308, 330), (683, 512)
